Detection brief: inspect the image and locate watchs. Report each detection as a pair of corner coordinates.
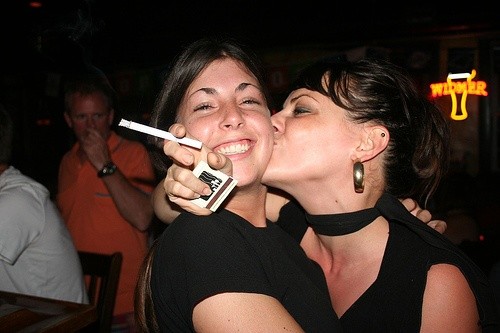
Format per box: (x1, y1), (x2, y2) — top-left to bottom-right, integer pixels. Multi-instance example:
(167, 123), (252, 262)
(98, 161), (118, 177)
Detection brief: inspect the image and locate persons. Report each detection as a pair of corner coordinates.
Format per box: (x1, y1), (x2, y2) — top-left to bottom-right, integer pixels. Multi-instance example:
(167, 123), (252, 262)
(132, 37), (447, 332)
(0, 105), (95, 304)
(153, 57), (484, 333)
(53, 66), (154, 332)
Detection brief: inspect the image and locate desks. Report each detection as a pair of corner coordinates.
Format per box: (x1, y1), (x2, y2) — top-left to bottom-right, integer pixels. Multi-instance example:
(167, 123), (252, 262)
(1, 290), (99, 333)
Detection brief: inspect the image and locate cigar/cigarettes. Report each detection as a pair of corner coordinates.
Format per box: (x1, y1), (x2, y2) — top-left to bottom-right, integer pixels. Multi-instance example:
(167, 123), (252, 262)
(119, 118), (202, 153)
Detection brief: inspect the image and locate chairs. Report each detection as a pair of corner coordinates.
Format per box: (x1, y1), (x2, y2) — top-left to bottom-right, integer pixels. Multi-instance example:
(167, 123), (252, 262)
(77, 250), (124, 333)
